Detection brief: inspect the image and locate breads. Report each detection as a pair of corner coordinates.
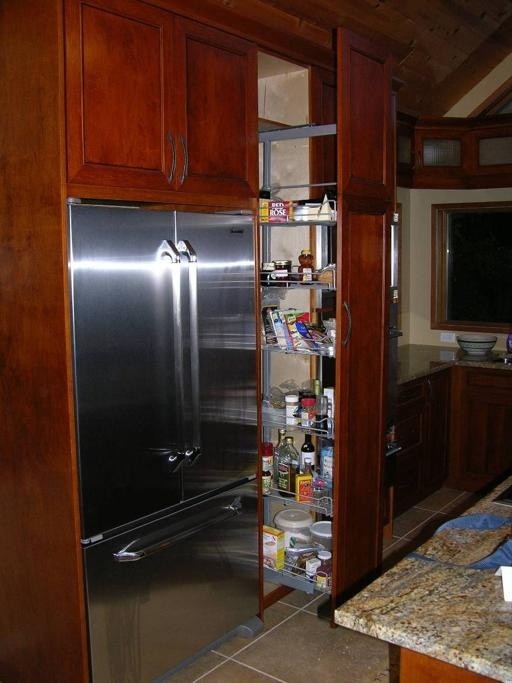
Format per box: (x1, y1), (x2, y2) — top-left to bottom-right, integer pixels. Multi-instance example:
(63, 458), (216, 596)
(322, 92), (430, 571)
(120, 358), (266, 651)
(317, 270), (337, 286)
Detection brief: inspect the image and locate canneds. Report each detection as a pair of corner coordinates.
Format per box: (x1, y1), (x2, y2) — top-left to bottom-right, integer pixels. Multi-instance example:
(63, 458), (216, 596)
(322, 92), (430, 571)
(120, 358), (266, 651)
(274, 260), (292, 285)
(261, 262), (276, 285)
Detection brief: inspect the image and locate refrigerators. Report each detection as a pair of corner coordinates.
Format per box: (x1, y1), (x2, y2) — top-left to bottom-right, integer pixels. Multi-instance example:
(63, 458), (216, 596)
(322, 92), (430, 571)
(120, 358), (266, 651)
(69, 201), (265, 682)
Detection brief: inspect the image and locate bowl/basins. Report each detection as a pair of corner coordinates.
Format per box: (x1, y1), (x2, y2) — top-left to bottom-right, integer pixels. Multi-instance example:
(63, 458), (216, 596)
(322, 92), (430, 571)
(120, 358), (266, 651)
(456, 334), (498, 356)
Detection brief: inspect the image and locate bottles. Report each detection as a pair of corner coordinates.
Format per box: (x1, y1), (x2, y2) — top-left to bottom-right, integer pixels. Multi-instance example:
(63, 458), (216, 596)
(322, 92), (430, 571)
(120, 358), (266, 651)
(262, 433), (333, 505)
(273, 260), (292, 287)
(283, 395), (329, 436)
(298, 250), (315, 286)
(262, 262), (277, 287)
(317, 550), (332, 574)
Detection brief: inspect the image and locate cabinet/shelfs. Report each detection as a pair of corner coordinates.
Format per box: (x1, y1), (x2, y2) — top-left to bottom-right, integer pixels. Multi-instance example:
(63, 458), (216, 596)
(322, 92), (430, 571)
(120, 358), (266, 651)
(261, 1), (398, 614)
(63, 0), (260, 207)
(399, 365), (511, 519)
